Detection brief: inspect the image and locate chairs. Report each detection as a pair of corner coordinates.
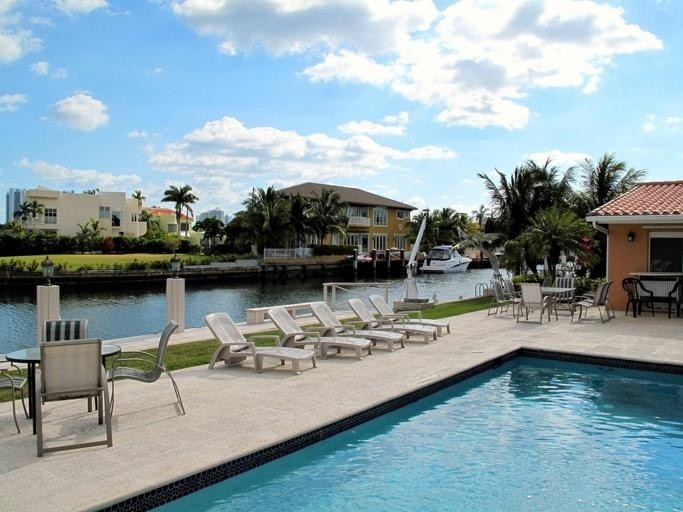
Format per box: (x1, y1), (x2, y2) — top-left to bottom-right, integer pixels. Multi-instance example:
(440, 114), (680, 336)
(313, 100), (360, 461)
(351, 298), (437, 345)
(621, 277), (655, 318)
(267, 305), (373, 361)
(106, 320), (185, 419)
(369, 294), (452, 337)
(667, 276), (683, 319)
(41, 319), (88, 342)
(485, 276), (615, 323)
(34, 338), (113, 458)
(311, 301), (406, 353)
(205, 312), (316, 375)
(0, 359), (29, 434)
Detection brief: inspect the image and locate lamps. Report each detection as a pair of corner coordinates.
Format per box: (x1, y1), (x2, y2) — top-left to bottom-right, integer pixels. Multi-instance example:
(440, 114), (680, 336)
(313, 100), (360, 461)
(627, 231), (636, 241)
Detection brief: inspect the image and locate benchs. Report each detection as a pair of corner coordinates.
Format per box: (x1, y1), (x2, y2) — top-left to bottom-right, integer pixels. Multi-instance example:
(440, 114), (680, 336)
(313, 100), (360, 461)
(246, 301), (313, 326)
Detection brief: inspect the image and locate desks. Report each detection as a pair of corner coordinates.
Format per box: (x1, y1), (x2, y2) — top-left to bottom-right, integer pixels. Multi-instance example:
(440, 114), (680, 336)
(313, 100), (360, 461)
(5, 343), (122, 436)
(629, 272), (683, 317)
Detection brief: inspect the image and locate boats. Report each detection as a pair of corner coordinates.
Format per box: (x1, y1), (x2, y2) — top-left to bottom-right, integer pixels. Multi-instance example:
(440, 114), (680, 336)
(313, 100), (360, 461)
(418, 243), (472, 275)
